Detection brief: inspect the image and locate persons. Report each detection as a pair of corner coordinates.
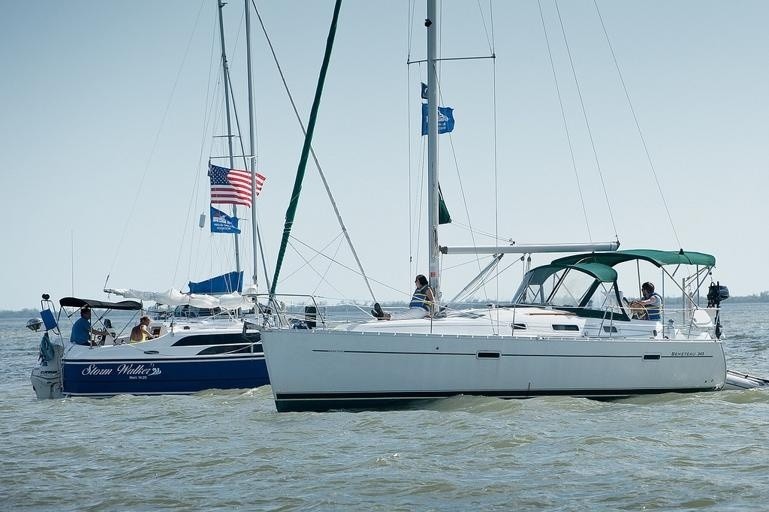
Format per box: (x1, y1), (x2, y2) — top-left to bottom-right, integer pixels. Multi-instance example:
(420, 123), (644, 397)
(626, 282), (663, 321)
(71, 309), (108, 346)
(130, 316), (155, 344)
(371, 275), (436, 320)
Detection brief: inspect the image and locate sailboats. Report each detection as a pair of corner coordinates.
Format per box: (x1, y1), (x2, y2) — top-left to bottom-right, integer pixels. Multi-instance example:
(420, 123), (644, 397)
(26, 0), (376, 398)
(260, 0), (769, 412)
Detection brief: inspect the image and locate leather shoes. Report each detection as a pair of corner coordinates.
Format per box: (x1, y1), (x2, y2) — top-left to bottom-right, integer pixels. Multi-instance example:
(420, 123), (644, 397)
(371, 303), (391, 321)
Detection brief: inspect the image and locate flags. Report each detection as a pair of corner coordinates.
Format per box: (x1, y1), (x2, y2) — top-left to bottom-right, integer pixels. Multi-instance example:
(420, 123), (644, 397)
(210, 163), (266, 234)
(421, 104), (455, 136)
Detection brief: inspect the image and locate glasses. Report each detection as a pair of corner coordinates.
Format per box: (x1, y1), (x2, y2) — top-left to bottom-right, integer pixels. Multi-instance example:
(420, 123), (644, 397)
(415, 279), (419, 283)
(642, 288), (645, 291)
